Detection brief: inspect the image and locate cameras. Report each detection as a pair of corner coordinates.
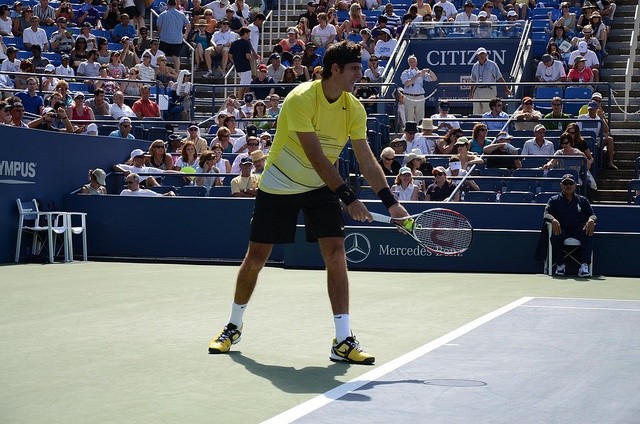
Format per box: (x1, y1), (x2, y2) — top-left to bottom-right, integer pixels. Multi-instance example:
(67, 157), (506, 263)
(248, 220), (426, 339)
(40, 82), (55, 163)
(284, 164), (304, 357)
(266, 139), (272, 146)
(127, 41), (132, 45)
(230, 99), (235, 103)
(49, 113), (59, 118)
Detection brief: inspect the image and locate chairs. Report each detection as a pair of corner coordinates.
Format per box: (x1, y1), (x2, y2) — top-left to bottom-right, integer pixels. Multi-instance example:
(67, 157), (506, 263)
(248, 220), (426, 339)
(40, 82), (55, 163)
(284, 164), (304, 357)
(338, 113), (600, 203)
(82, 154), (239, 195)
(15, 198), (70, 262)
(37, 199), (89, 262)
(0, 0), (175, 139)
(543, 220), (596, 276)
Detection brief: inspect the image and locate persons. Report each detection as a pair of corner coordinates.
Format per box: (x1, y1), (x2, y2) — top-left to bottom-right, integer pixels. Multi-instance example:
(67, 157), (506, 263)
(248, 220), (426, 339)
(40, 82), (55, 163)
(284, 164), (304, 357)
(424, 166), (461, 202)
(278, 27), (306, 52)
(433, 0), (458, 21)
(566, 56), (594, 92)
(417, 118), (439, 152)
(249, 64), (275, 99)
(396, 87), (405, 131)
(544, 173), (598, 278)
(224, 116), (244, 147)
(194, 1), (265, 77)
(578, 92), (609, 123)
(509, 0), (537, 20)
(469, 124), (492, 157)
(166, 135), (185, 153)
(520, 124), (556, 155)
(174, 141), (200, 170)
(577, 1), (596, 29)
(482, 98), (511, 132)
(297, 2), (319, 31)
(422, 14), (432, 21)
(210, 126), (235, 153)
(400, 121), (432, 155)
(542, 133), (592, 175)
(543, 97), (575, 130)
(444, 157), (480, 191)
(400, 55), (438, 123)
(480, 2), (499, 38)
(374, 28), (398, 59)
(430, 101), (460, 130)
(364, 55), (384, 83)
(469, 47), (511, 115)
(291, 55), (311, 82)
(112, 149), (184, 186)
(259, 131), (272, 154)
(500, 10), (524, 38)
(249, 101), (274, 130)
(378, 147), (401, 176)
(395, 14), (414, 37)
(250, 150), (267, 174)
(404, 152), (426, 189)
(569, 25), (602, 53)
(208, 42), (414, 364)
(477, 11), (493, 38)
(147, 140), (174, 170)
(279, 69), (300, 96)
(484, 131), (521, 168)
(356, 77), (380, 107)
(80, 169), (109, 194)
(454, 0), (478, 33)
(208, 113), (228, 134)
(495, 0), (513, 19)
(407, 0), (433, 16)
(268, 54), (287, 98)
(370, 16), (396, 39)
(215, 94), (246, 128)
(180, 124), (207, 153)
(119, 173), (176, 196)
(300, 42), (323, 80)
(241, 92), (253, 118)
(374, 3), (402, 34)
(230, 157), (258, 197)
(544, 2), (576, 37)
(409, 6), (423, 22)
(311, 12), (337, 46)
(564, 123), (594, 164)
(231, 136), (260, 173)
(579, 102), (618, 169)
(568, 41), (600, 91)
(317, 6), (326, 12)
(548, 41), (567, 70)
(450, 137), (483, 171)
(327, 7), (350, 41)
(433, 127), (464, 153)
(510, 96), (543, 131)
(433, 6), (447, 21)
(357, 28), (375, 59)
(549, 25), (572, 62)
(348, 3), (367, 33)
(267, 94), (281, 117)
(295, 17), (310, 42)
(267, 44), (294, 67)
(194, 151), (222, 186)
(0, 0), (191, 139)
(589, 11), (610, 56)
(232, 124), (262, 153)
(536, 54), (567, 82)
(210, 144), (231, 181)
(390, 167), (420, 201)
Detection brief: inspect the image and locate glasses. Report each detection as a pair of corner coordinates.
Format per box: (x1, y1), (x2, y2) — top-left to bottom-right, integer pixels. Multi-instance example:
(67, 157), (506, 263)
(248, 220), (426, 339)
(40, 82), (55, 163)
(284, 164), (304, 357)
(361, 32), (366, 35)
(12, 109), (25, 112)
(136, 156), (145, 158)
(224, 134), (231, 138)
(479, 129), (488, 132)
(392, 144), (404, 147)
(59, 88), (68, 91)
(24, 10), (33, 13)
(153, 146), (164, 148)
(76, 97), (84, 99)
(553, 103), (561, 107)
(568, 132), (575, 134)
(498, 104), (503, 107)
(317, 18), (323, 20)
(562, 181), (574, 185)
(299, 20), (305, 24)
(544, 72), (552, 77)
(2, 109), (10, 112)
(455, 145), (465, 147)
(370, 59), (378, 61)
(161, 59), (166, 61)
(466, 6), (472, 7)
(248, 143), (259, 146)
(286, 71), (292, 73)
(424, 18), (431, 21)
(206, 13), (212, 16)
(123, 18), (129, 19)
(383, 156), (395, 161)
(125, 181), (136, 185)
(141, 33), (147, 34)
(223, 23), (230, 25)
(121, 125), (131, 128)
(434, 173), (442, 176)
(189, 128), (198, 131)
(272, 99), (279, 101)
(206, 157), (215, 160)
(220, 116), (228, 118)
(127, 73), (133, 74)
(102, 43), (108, 45)
(257, 104), (263, 106)
(592, 99), (601, 102)
(214, 151), (222, 154)
(229, 120), (237, 121)
(28, 83), (37, 85)
(486, 6), (493, 8)
(561, 141), (570, 145)
(564, 6), (569, 8)
(143, 55), (150, 58)
(525, 103), (532, 105)
(226, 10), (233, 13)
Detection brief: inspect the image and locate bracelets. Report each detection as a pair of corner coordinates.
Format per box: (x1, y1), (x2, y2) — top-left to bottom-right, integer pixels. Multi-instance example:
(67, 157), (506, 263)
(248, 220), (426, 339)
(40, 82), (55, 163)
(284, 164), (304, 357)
(335, 184), (359, 207)
(428, 69), (430, 73)
(377, 187), (399, 209)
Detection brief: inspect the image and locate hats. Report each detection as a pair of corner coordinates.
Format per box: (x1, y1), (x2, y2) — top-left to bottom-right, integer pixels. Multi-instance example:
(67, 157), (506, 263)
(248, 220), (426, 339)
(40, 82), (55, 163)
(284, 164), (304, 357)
(522, 96), (532, 103)
(588, 102), (597, 110)
(44, 64), (56, 72)
(245, 94), (253, 103)
(404, 152), (425, 163)
(392, 87), (403, 98)
(112, 1), (118, 6)
(305, 42), (316, 48)
(13, 1), (22, 6)
(92, 168), (106, 186)
(404, 123), (418, 132)
(560, 2), (571, 10)
(477, 47), (487, 55)
(131, 149), (146, 161)
(188, 124), (199, 129)
(119, 117), (131, 129)
(314, 66), (323, 73)
(227, 6), (235, 12)
(260, 131), (272, 138)
(361, 76), (370, 82)
(274, 45), (282, 52)
(247, 124), (257, 137)
(478, 11), (488, 18)
(221, 0), (228, 5)
(218, 111), (227, 117)
(360, 28), (372, 39)
(21, 5), (33, 14)
(293, 55), (301, 59)
(270, 53), (280, 58)
(61, 54), (70, 60)
(42, 107), (56, 116)
(7, 47), (19, 53)
(464, 1), (474, 9)
(574, 57), (587, 63)
(432, 166), (447, 175)
(399, 167), (412, 176)
(579, 41), (587, 53)
(507, 11), (517, 17)
(57, 17), (67, 22)
(592, 92), (603, 99)
(74, 92), (85, 98)
(0, 5), (12, 11)
(194, 19), (210, 26)
(82, 22), (94, 30)
(582, 25), (593, 35)
(111, 52), (120, 56)
(381, 28), (392, 39)
(258, 64), (268, 73)
(454, 137), (469, 145)
(561, 174), (575, 184)
(121, 36), (130, 42)
(228, 94), (236, 99)
(582, 0), (596, 8)
(0, 101), (12, 110)
(497, 133), (513, 140)
(410, 7), (417, 13)
(440, 101), (449, 111)
(184, 70), (192, 75)
(449, 158), (461, 171)
(250, 150), (267, 162)
(534, 124), (547, 132)
(221, 17), (229, 22)
(416, 119), (438, 130)
(54, 102), (65, 110)
(590, 11), (602, 18)
(240, 158), (253, 165)
(31, 44), (41, 50)
(287, 27), (298, 34)
(542, 54), (551, 62)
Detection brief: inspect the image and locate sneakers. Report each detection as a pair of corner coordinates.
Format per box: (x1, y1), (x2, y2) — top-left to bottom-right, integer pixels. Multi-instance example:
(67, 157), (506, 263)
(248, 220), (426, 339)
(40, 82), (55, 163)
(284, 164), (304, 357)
(209, 323), (243, 353)
(203, 71), (214, 77)
(555, 264), (566, 275)
(609, 162), (618, 169)
(578, 263), (589, 277)
(220, 70), (226, 78)
(330, 329), (375, 365)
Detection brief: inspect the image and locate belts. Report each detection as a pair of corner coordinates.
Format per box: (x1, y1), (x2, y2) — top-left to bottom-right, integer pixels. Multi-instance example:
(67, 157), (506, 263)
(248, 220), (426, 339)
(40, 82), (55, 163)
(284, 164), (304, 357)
(405, 93), (425, 97)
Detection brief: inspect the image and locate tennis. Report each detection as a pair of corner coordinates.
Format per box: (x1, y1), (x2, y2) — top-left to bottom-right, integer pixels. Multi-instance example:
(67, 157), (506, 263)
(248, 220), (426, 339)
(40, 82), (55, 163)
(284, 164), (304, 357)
(403, 217), (414, 232)
